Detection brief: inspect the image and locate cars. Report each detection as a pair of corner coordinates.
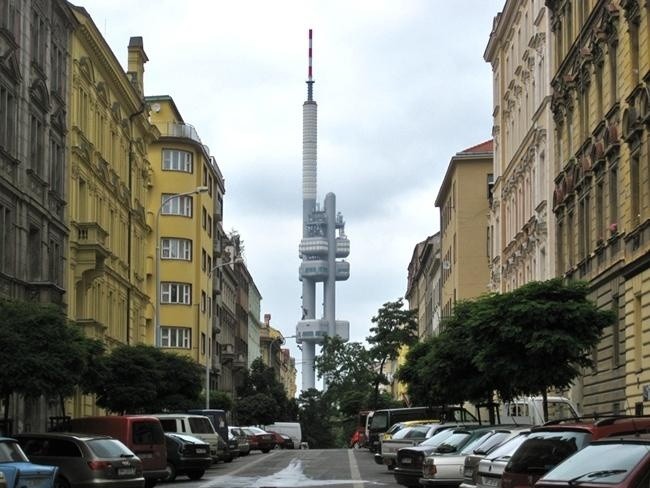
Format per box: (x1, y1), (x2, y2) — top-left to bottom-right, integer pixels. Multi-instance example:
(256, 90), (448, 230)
(0, 436), (59, 488)
(265, 431), (294, 450)
(10, 431), (147, 488)
(229, 427), (251, 457)
(243, 425), (276, 454)
(350, 396), (650, 488)
(163, 433), (213, 480)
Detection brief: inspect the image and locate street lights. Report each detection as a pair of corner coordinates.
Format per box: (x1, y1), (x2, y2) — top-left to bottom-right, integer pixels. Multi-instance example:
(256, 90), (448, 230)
(206, 258), (243, 410)
(155, 185), (208, 349)
(269, 335), (298, 366)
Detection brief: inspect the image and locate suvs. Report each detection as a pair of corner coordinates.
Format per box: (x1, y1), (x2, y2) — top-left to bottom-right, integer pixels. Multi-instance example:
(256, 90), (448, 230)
(44, 415), (170, 483)
(156, 414), (219, 463)
